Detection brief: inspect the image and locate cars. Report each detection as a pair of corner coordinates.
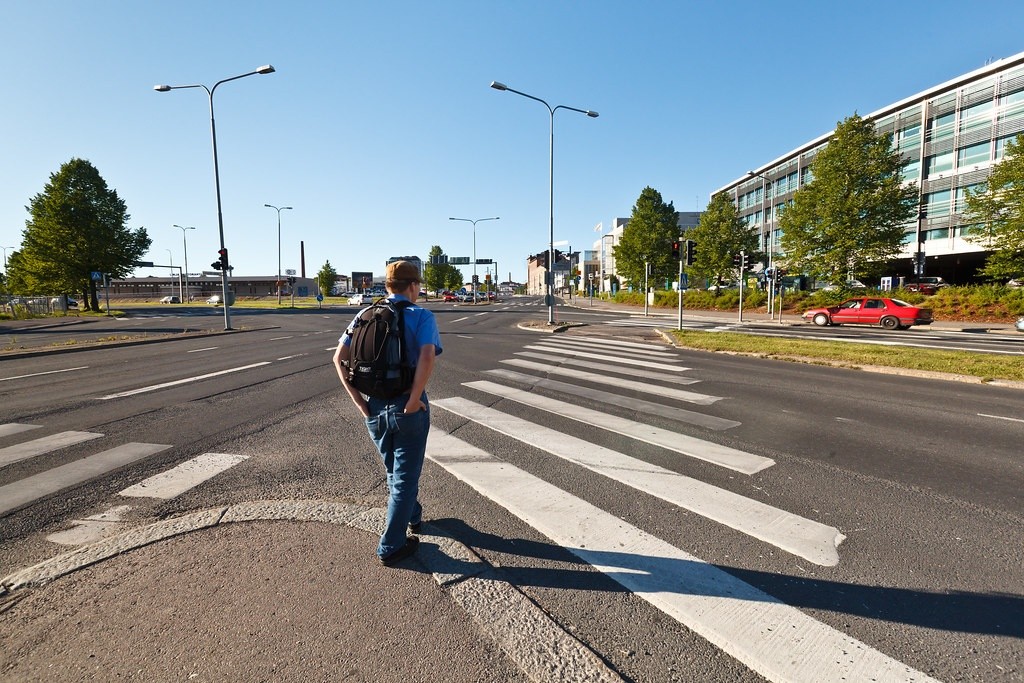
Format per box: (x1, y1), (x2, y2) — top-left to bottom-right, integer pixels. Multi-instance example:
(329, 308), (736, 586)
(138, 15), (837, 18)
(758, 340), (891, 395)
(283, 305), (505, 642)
(206, 296), (223, 305)
(905, 277), (949, 295)
(801, 298), (934, 330)
(6, 296), (78, 307)
(1014, 316), (1024, 331)
(342, 289), (496, 306)
(823, 280), (866, 291)
(159, 296), (180, 304)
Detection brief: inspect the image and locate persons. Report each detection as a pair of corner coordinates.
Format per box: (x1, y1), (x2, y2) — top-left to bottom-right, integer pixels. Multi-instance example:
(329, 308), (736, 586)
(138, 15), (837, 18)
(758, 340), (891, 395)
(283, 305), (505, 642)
(334, 260), (444, 566)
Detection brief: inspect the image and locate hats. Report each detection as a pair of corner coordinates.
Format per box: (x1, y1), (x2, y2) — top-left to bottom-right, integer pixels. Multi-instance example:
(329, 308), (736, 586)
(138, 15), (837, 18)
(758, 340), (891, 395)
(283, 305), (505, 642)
(386, 260), (427, 286)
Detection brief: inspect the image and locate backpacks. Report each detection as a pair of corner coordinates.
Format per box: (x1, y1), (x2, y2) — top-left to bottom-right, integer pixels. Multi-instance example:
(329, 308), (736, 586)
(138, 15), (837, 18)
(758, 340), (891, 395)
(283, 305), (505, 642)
(342, 298), (416, 398)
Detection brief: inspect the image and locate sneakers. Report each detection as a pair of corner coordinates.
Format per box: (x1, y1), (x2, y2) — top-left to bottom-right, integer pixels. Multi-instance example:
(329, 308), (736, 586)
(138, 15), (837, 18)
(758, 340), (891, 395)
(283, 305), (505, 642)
(407, 509), (423, 532)
(377, 535), (421, 566)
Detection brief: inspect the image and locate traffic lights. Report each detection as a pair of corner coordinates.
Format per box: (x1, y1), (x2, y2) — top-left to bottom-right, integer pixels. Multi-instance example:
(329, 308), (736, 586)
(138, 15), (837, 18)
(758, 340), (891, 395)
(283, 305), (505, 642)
(767, 270), (773, 279)
(218, 248), (228, 269)
(672, 241), (680, 261)
(687, 240), (697, 265)
(776, 268), (781, 283)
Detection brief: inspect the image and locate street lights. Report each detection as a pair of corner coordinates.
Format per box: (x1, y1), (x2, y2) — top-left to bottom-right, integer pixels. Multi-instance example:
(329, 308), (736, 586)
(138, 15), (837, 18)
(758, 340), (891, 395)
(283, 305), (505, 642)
(449, 217), (501, 304)
(490, 81), (599, 326)
(264, 204), (293, 305)
(4, 246), (15, 287)
(747, 171), (774, 313)
(173, 225), (195, 304)
(153, 64), (276, 330)
